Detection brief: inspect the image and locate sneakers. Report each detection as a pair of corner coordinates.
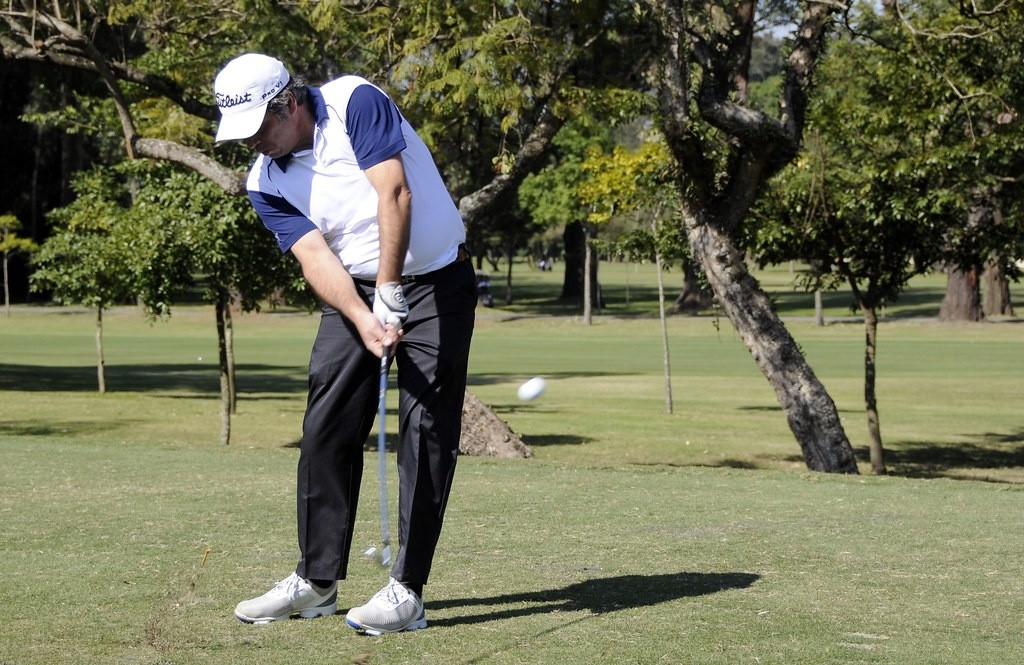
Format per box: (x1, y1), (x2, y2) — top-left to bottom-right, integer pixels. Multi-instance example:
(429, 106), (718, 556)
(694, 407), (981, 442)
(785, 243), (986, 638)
(345, 576), (428, 636)
(234, 570), (338, 625)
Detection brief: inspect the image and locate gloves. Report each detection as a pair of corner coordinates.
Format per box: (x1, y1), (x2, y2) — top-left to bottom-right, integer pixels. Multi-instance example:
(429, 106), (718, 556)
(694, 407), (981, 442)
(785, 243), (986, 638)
(372, 281), (409, 330)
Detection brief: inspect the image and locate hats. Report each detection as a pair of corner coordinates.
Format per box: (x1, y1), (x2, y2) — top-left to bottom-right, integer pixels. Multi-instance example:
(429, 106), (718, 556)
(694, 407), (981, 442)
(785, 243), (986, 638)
(213, 53), (291, 143)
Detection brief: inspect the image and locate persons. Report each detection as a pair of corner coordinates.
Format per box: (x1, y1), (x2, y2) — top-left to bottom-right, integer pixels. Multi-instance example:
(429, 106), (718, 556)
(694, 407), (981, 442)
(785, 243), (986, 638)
(213, 54), (480, 637)
(539, 257), (552, 271)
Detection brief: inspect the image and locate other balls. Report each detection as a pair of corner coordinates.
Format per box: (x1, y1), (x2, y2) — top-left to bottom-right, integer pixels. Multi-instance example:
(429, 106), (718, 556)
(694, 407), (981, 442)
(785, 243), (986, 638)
(516, 373), (548, 404)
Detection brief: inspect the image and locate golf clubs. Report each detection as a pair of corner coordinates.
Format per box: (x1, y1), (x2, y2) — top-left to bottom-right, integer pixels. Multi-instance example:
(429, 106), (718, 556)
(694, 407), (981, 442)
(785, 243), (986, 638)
(365, 339), (393, 570)
